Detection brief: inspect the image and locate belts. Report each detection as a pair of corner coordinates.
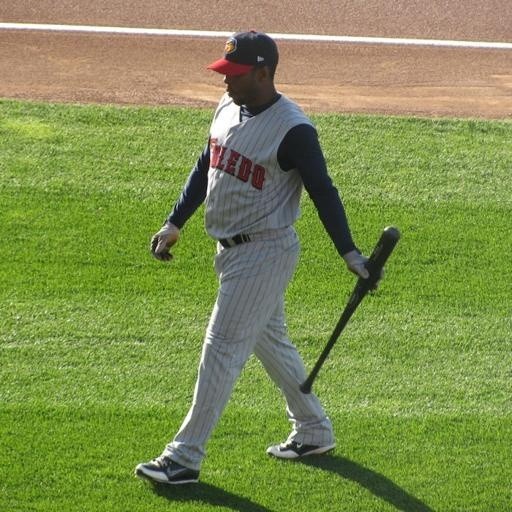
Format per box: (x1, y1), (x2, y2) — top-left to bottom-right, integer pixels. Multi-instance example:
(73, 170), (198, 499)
(218, 232), (251, 250)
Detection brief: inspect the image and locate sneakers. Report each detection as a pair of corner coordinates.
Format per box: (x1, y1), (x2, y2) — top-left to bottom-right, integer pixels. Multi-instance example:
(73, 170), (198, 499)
(134, 454), (201, 486)
(266, 431), (337, 461)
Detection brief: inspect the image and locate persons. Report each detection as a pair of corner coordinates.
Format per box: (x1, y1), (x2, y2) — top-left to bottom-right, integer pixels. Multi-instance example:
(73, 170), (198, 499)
(133, 28), (386, 488)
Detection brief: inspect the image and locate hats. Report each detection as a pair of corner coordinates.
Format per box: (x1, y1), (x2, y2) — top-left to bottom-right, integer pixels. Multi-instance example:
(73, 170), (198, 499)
(205, 29), (280, 78)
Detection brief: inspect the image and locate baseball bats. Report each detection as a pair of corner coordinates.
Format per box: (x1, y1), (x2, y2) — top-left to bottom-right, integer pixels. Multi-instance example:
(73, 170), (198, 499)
(299, 227), (400, 394)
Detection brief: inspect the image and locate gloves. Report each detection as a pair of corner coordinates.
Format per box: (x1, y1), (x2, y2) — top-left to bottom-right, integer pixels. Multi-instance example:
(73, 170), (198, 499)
(149, 219), (182, 262)
(340, 248), (386, 292)
